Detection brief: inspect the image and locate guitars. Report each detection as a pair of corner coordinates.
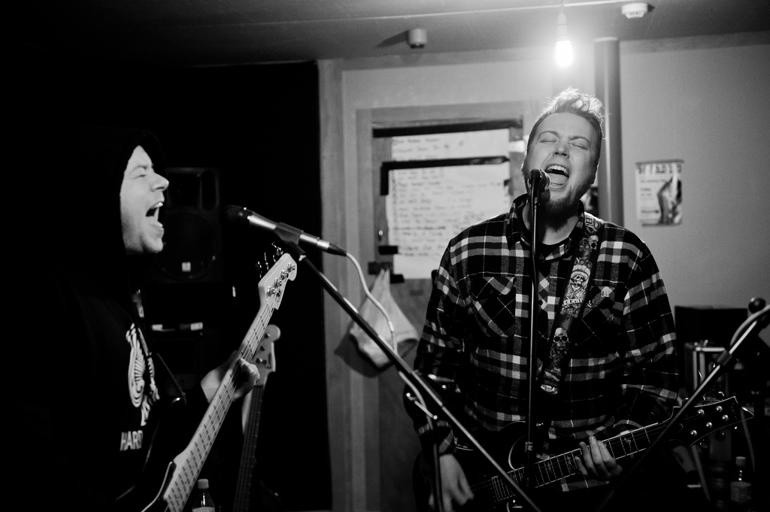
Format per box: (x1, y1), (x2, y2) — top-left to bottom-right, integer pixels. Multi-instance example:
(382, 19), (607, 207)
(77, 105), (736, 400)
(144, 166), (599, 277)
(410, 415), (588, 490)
(227, 326), (280, 512)
(112, 240), (298, 512)
(413, 387), (755, 512)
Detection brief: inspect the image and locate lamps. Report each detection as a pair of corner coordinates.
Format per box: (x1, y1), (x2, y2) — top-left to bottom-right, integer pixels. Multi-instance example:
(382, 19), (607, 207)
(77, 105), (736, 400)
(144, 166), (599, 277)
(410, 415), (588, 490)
(554, 1), (574, 66)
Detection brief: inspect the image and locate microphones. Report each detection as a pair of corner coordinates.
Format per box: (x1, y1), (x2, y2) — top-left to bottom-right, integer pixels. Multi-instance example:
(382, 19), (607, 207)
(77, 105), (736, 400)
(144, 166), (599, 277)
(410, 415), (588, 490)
(528, 167), (550, 191)
(219, 204), (347, 255)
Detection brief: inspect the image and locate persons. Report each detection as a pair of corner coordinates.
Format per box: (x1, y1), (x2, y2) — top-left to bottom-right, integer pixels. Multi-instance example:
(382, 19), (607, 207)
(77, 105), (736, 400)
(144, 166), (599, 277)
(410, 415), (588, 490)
(0, 144), (261, 512)
(403, 98), (683, 510)
